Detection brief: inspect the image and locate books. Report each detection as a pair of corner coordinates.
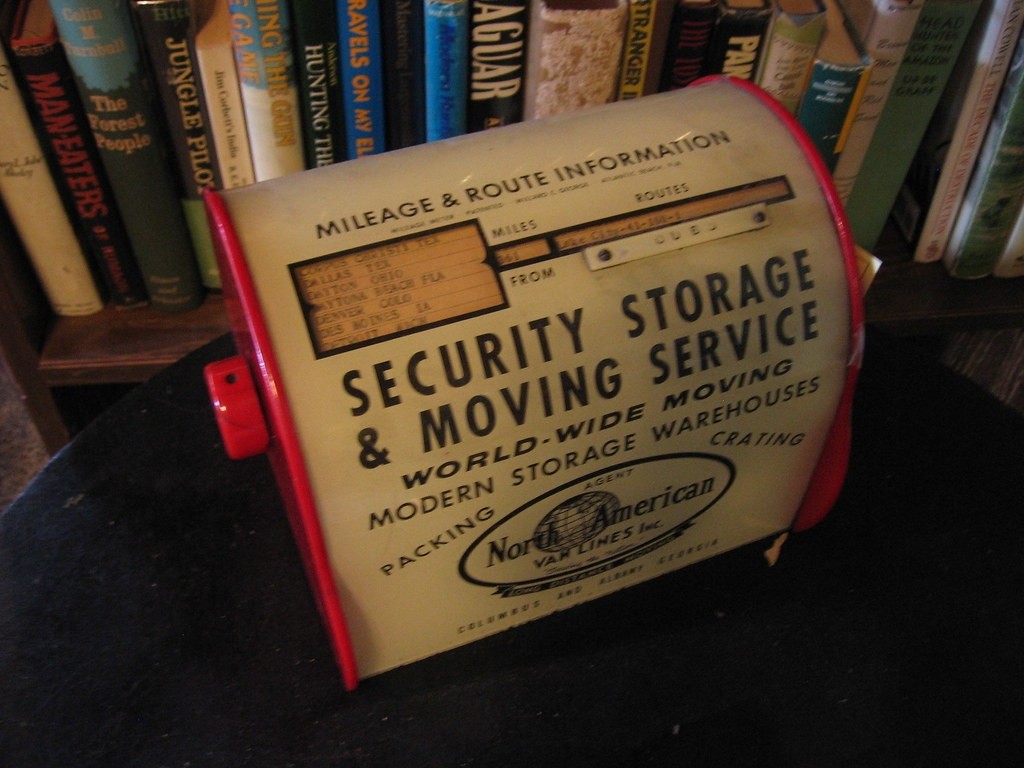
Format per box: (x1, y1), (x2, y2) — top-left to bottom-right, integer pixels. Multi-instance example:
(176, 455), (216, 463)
(0, 0), (1024, 320)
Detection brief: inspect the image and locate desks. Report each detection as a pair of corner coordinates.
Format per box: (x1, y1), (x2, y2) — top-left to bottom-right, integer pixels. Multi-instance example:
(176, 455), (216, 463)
(0, 329), (1024, 768)
